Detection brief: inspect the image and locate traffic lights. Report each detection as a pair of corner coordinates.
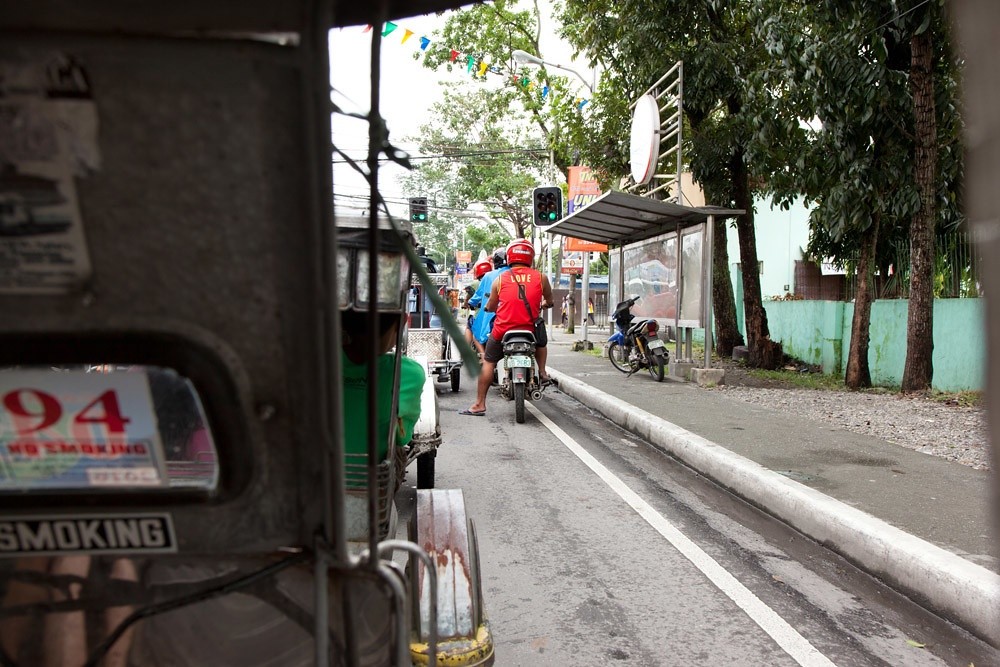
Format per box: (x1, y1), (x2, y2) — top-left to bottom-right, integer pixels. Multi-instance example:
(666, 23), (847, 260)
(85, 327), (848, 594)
(532, 187), (563, 227)
(409, 197), (428, 223)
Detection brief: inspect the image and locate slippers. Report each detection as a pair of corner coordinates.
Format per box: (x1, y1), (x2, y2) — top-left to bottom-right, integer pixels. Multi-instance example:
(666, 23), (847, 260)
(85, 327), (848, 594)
(459, 408), (486, 416)
(541, 376), (551, 385)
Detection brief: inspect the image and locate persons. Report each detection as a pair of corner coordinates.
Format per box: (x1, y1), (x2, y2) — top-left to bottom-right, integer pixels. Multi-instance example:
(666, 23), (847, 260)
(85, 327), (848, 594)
(460, 238), (555, 417)
(341, 293), (428, 560)
(588, 298), (596, 325)
(559, 294), (576, 327)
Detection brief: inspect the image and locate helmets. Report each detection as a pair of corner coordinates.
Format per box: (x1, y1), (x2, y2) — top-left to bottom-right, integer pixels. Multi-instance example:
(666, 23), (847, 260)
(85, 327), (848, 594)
(506, 238), (535, 269)
(473, 261), (492, 279)
(493, 247), (508, 269)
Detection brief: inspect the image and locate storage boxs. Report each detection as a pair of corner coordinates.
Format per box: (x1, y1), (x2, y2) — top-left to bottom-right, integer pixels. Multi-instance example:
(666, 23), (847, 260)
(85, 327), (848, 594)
(795, 260), (896, 300)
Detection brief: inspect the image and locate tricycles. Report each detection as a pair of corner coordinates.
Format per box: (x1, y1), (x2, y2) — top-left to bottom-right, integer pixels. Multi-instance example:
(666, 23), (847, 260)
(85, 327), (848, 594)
(392, 242), (463, 392)
(2, 4), (496, 667)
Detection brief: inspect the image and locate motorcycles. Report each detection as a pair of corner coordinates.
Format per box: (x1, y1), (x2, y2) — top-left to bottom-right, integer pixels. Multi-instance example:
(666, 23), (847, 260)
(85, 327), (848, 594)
(491, 329), (558, 423)
(607, 294), (670, 382)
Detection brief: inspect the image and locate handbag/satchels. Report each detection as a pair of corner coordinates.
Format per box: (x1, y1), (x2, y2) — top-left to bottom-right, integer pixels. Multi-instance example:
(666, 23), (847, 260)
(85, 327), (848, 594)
(534, 317), (547, 347)
